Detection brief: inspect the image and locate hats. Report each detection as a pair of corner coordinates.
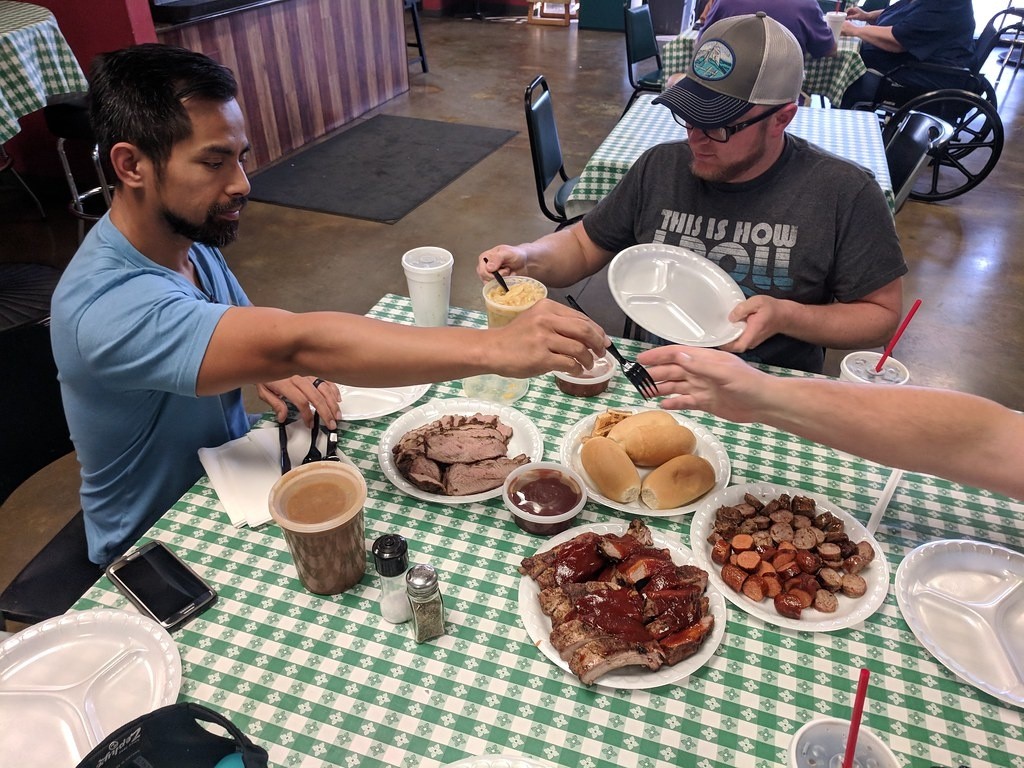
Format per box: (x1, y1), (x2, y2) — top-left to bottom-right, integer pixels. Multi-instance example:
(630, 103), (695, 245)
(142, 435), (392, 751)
(652, 12), (804, 129)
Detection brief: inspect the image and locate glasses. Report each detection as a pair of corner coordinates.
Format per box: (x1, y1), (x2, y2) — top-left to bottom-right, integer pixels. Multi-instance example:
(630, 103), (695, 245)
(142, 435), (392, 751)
(671, 104), (782, 143)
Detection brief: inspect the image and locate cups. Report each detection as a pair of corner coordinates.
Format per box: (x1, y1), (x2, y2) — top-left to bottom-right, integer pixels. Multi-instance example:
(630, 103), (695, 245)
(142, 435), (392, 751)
(788, 718), (902, 768)
(481, 275), (549, 329)
(838, 351), (910, 385)
(268, 460), (367, 595)
(825, 11), (847, 44)
(400, 245), (455, 328)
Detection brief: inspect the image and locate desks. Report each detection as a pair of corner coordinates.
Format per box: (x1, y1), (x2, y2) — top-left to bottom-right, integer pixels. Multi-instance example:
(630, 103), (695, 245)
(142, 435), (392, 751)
(662, 15), (867, 105)
(0, 0), (90, 206)
(564, 93), (895, 232)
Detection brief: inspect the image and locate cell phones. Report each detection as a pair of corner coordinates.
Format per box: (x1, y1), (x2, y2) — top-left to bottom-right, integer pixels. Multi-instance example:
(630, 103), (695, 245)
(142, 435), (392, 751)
(105, 539), (218, 636)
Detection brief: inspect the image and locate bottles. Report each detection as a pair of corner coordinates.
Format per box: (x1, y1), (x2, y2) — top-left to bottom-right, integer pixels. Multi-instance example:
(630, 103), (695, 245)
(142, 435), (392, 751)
(405, 563), (446, 645)
(371, 533), (411, 624)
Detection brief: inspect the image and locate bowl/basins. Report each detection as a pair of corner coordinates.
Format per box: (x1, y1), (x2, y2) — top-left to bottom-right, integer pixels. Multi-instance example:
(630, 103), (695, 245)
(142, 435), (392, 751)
(501, 462), (588, 536)
(552, 348), (617, 397)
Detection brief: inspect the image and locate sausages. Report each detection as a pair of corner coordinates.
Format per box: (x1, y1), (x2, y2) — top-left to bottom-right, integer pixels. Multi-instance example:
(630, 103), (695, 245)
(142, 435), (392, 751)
(707, 494), (874, 620)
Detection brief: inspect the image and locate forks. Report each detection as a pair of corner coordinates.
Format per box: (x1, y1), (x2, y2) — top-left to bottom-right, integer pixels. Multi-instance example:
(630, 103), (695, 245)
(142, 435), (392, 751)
(565, 294), (660, 403)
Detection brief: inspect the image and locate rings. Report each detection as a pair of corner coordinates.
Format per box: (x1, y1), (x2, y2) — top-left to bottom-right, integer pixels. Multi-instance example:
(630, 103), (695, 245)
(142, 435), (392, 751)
(313, 376), (326, 388)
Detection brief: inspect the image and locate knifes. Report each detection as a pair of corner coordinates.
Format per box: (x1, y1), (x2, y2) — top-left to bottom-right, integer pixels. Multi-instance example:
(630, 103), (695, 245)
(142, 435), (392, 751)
(279, 421), (292, 476)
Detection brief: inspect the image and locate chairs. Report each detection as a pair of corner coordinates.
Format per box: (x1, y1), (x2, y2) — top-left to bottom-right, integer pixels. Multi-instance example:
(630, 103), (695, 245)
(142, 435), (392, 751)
(0, 66), (308, 626)
(523, 0), (953, 335)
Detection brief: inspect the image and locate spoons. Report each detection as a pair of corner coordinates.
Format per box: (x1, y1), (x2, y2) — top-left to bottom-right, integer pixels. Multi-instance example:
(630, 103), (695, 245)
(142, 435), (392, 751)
(301, 409), (322, 465)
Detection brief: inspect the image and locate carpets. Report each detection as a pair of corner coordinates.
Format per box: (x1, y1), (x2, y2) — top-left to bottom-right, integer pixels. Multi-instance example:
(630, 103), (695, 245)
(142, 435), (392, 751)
(248, 114), (520, 225)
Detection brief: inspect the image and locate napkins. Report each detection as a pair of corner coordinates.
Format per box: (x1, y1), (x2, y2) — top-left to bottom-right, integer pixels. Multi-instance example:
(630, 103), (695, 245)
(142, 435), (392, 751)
(196, 419), (365, 527)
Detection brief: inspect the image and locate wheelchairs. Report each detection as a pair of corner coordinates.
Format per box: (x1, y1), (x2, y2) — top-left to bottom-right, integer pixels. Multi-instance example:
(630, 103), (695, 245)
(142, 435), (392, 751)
(848, 6), (1024, 204)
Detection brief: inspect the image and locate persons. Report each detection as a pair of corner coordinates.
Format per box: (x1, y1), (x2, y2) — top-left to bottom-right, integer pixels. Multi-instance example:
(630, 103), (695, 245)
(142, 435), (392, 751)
(629, 344), (1024, 502)
(51, 44), (599, 575)
(686, 0), (976, 131)
(478, 10), (909, 372)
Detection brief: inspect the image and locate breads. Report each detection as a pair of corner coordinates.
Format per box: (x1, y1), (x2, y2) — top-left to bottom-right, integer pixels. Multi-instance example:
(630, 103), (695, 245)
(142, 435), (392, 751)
(624, 423), (696, 466)
(641, 454), (715, 510)
(606, 411), (678, 444)
(580, 436), (642, 502)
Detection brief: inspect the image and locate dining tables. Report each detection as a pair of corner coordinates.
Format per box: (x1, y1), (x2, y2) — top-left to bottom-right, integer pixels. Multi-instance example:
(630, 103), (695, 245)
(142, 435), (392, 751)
(64, 295), (1024, 768)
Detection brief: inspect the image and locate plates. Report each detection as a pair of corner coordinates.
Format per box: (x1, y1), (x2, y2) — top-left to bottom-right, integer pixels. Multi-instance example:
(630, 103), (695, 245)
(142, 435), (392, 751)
(442, 754), (554, 768)
(379, 398), (545, 504)
(690, 482), (890, 633)
(894, 539), (1024, 708)
(0, 609), (183, 768)
(518, 522), (727, 690)
(308, 382), (432, 420)
(560, 406), (732, 516)
(607, 243), (748, 347)
